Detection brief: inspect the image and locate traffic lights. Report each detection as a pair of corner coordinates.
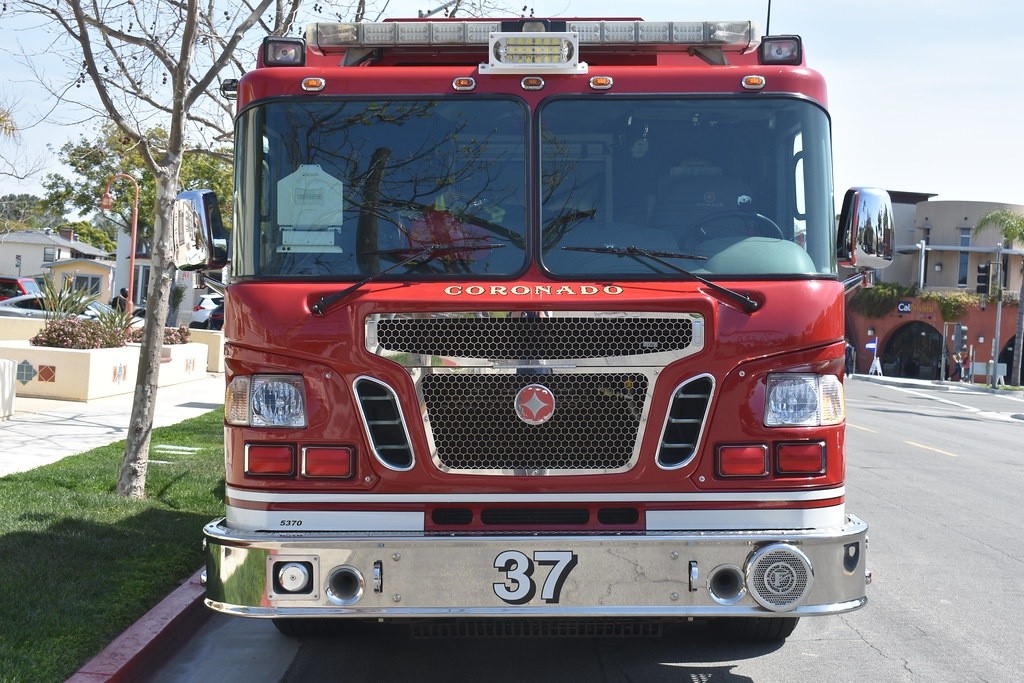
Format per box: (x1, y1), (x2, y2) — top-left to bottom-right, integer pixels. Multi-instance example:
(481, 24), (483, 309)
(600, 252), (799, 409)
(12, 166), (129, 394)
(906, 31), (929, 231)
(976, 260), (993, 296)
(951, 323), (968, 355)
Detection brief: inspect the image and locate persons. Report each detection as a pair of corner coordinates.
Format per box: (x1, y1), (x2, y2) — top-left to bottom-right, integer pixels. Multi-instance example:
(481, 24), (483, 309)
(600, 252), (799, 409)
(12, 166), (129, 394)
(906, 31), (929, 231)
(947, 350), (974, 383)
(112, 288), (129, 312)
(845, 339), (852, 378)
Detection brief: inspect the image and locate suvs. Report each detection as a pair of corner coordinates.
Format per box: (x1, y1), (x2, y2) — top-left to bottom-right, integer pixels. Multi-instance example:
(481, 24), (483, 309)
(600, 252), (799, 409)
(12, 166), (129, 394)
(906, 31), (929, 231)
(0, 274), (44, 304)
(187, 271), (226, 331)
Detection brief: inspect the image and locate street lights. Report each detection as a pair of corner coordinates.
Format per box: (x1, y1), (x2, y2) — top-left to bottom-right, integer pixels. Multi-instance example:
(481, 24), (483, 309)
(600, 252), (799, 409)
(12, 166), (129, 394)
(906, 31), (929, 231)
(99, 174), (141, 335)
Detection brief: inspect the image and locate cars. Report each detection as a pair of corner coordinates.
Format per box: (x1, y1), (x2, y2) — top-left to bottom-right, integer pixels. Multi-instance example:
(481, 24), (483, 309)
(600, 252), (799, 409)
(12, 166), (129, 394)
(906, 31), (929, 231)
(0, 290), (144, 332)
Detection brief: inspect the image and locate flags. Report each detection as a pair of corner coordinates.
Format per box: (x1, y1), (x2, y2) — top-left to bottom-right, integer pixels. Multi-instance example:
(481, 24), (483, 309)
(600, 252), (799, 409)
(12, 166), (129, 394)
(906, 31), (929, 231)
(864, 338), (876, 352)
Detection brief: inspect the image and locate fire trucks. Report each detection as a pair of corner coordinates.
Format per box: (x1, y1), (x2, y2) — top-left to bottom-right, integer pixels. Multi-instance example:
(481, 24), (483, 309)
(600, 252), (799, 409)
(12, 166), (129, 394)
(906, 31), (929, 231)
(163, 14), (898, 644)
